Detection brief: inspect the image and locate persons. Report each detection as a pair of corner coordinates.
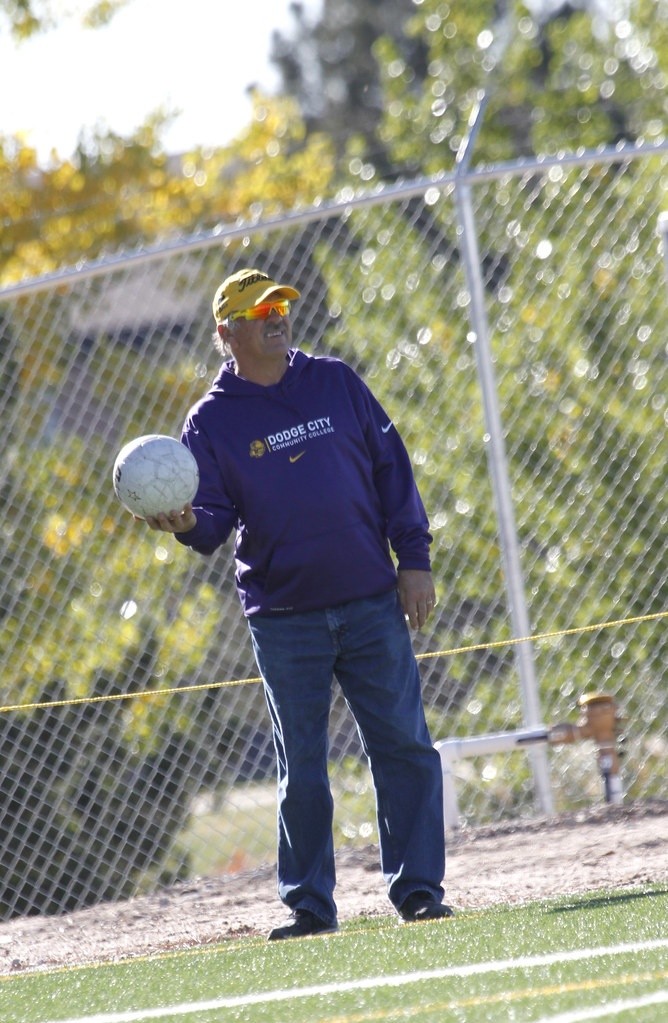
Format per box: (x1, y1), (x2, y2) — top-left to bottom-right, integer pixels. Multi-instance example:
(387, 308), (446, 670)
(131, 268), (452, 941)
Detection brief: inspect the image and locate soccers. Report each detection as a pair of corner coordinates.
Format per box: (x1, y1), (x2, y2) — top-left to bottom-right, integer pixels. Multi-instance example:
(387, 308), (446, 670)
(111, 432), (202, 518)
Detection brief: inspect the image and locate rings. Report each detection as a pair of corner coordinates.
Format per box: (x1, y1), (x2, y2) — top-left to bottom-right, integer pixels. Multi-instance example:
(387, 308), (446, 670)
(427, 600), (433, 604)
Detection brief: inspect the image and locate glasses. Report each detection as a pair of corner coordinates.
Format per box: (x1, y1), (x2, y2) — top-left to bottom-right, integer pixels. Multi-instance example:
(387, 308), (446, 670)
(228, 298), (292, 323)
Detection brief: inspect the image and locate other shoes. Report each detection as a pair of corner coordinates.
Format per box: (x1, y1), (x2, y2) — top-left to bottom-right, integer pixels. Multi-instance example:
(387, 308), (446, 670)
(397, 892), (454, 922)
(268, 907), (338, 941)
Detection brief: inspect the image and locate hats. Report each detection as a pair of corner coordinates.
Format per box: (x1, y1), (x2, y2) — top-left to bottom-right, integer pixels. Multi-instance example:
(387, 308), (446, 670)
(211, 268), (301, 323)
